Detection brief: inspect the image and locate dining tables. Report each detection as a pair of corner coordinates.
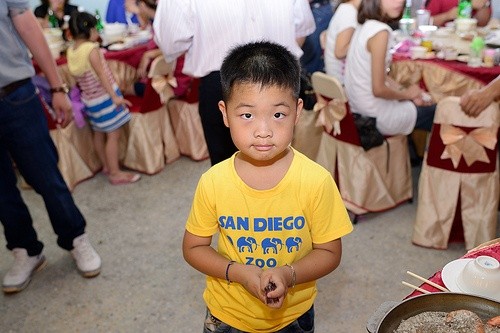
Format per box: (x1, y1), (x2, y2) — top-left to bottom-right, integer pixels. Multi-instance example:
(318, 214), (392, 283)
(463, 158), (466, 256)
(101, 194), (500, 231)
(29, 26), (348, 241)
(30, 24), (159, 92)
(390, 16), (500, 103)
(404, 236), (500, 302)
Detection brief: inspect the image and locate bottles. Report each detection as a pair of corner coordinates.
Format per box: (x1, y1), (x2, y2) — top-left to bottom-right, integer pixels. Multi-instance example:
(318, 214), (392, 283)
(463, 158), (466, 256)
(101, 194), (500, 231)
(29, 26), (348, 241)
(47, 7), (61, 27)
(402, 0), (412, 18)
(94, 9), (104, 32)
(457, 0), (472, 17)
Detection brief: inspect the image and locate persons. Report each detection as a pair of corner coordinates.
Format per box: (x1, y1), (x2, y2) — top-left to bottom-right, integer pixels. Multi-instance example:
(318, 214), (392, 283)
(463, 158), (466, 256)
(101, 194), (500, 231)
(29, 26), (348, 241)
(33, 0), (500, 185)
(182, 41), (353, 333)
(0, 0), (101, 293)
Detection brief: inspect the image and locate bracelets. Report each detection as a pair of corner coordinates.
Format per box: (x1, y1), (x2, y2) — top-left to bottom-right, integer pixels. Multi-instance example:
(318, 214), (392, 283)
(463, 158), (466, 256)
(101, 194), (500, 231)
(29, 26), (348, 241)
(226, 261), (236, 282)
(285, 264), (296, 288)
(399, 86), (404, 91)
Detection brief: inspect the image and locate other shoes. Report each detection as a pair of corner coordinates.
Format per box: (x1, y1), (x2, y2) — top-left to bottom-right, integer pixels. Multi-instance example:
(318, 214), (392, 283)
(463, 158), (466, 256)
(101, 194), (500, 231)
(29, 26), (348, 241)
(110, 174), (141, 184)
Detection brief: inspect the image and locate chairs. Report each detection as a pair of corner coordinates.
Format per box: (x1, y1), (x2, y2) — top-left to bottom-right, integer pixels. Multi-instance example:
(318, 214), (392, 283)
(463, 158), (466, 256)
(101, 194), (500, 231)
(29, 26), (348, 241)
(411, 94), (500, 249)
(168, 54), (209, 161)
(110, 52), (181, 176)
(15, 86), (101, 193)
(309, 71), (415, 224)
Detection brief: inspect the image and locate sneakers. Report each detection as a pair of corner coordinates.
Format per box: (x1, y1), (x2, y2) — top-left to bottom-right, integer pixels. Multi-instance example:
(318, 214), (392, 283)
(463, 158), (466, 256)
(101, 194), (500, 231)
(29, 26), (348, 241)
(70, 233), (101, 276)
(2, 249), (47, 291)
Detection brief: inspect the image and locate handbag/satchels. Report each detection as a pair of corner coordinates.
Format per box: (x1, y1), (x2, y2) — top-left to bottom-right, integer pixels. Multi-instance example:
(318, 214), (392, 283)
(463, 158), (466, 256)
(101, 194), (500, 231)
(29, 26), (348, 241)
(351, 110), (385, 150)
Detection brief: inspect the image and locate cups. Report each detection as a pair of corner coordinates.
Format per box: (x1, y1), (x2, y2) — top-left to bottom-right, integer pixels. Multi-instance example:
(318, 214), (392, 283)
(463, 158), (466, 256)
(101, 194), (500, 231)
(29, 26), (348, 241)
(398, 9), (499, 66)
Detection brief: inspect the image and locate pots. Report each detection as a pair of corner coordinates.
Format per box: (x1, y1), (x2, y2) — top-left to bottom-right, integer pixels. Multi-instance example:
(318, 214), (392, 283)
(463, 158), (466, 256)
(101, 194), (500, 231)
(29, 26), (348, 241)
(365, 292), (500, 333)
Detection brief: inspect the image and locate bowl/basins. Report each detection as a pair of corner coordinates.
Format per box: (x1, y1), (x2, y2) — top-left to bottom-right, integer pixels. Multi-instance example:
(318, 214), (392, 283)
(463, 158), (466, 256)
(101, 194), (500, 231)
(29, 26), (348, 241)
(457, 255), (500, 294)
(42, 29), (63, 42)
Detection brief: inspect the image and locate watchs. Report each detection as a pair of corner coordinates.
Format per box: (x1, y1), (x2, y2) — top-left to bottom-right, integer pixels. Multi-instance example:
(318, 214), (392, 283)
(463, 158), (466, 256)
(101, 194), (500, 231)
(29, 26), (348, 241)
(50, 84), (70, 93)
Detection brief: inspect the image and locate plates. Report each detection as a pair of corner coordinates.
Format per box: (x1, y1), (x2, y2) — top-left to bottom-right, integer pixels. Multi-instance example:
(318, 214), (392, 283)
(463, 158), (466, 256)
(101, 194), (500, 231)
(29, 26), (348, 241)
(441, 258), (500, 301)
(401, 270), (449, 293)
